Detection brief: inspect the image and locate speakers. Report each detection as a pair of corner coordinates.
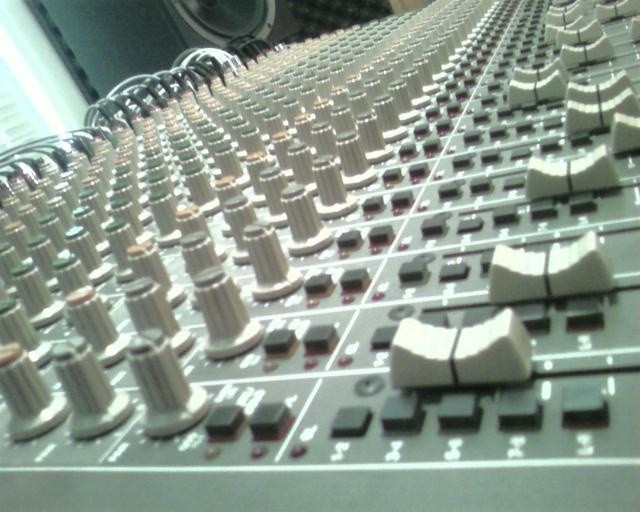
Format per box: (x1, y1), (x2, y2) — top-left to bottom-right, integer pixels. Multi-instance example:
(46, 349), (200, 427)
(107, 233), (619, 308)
(1, 0), (395, 107)
